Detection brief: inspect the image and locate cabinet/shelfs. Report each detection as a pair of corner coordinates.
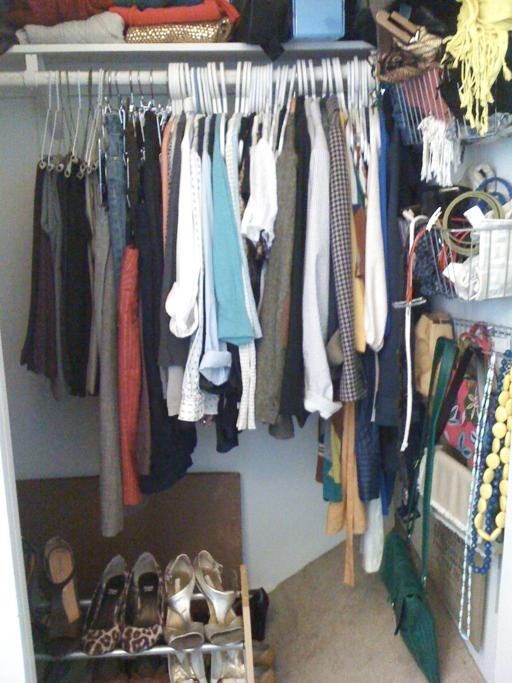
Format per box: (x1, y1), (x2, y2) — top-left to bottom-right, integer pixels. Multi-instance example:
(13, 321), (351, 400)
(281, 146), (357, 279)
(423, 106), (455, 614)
(28, 564), (254, 683)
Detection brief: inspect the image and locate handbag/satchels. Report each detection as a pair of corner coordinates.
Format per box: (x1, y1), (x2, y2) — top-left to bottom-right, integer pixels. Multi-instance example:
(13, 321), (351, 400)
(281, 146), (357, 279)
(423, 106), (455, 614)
(439, 323), (497, 470)
(379, 532), (439, 683)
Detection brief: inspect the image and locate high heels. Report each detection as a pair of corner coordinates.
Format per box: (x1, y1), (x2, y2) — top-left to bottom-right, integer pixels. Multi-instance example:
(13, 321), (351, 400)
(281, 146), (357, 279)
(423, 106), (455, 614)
(21, 536), (275, 683)
